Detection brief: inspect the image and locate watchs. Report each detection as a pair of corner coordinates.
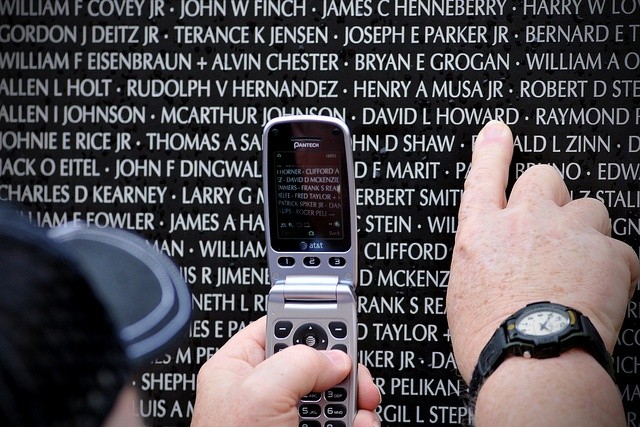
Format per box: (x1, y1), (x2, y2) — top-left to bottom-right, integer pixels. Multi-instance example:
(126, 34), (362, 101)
(468, 299), (615, 411)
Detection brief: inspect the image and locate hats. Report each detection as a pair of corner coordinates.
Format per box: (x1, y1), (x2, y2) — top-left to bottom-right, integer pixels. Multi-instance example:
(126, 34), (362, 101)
(1, 207), (193, 426)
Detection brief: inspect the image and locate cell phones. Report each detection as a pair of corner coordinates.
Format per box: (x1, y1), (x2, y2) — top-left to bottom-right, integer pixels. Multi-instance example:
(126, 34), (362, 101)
(263, 115), (357, 427)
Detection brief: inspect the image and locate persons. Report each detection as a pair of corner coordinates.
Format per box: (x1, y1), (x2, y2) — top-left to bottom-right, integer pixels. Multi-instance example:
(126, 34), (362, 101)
(1, 120), (640, 426)
